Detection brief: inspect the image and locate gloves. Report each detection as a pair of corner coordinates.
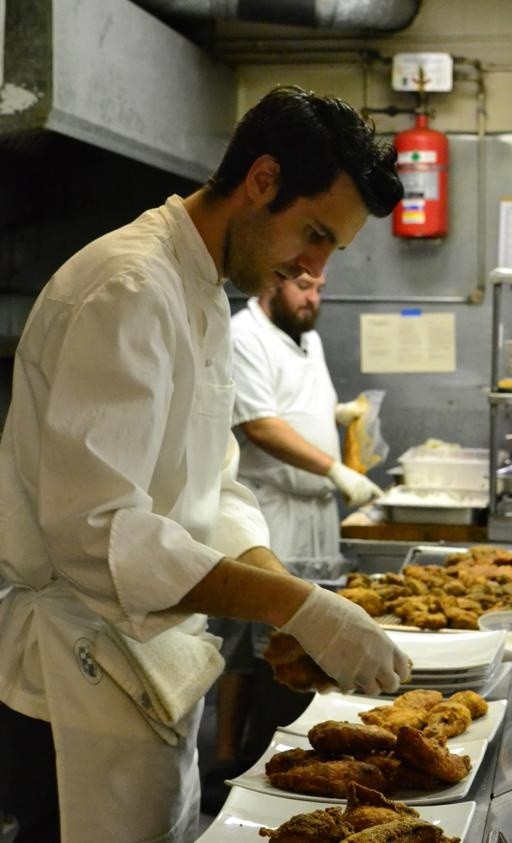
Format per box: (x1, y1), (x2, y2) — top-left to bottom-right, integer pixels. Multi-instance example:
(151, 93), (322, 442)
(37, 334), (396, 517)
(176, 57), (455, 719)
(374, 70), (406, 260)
(325, 460), (383, 507)
(334, 400), (362, 428)
(275, 582), (412, 694)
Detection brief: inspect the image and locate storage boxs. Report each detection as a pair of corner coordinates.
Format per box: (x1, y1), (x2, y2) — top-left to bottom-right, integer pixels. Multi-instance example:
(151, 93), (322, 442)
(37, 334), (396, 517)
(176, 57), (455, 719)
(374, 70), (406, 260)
(398, 447), (506, 490)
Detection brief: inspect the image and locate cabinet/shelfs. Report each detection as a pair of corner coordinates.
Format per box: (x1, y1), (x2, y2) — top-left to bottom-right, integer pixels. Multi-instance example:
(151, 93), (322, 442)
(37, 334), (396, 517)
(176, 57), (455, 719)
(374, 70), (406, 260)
(486, 271), (512, 542)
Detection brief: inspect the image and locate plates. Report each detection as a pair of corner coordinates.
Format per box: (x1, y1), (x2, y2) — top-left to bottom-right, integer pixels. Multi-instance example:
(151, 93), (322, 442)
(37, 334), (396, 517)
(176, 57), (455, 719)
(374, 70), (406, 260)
(225, 731), (487, 803)
(387, 629), (512, 699)
(280, 692), (511, 742)
(197, 786), (477, 842)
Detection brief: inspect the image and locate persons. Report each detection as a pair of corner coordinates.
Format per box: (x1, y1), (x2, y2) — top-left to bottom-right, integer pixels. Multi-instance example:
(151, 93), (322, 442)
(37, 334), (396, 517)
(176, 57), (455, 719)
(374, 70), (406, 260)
(0, 83), (412, 843)
(212, 269), (385, 786)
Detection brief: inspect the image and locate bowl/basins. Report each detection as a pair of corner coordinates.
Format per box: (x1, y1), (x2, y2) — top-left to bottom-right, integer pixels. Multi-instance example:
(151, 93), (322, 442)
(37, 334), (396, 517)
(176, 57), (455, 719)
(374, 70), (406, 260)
(478, 610), (512, 661)
